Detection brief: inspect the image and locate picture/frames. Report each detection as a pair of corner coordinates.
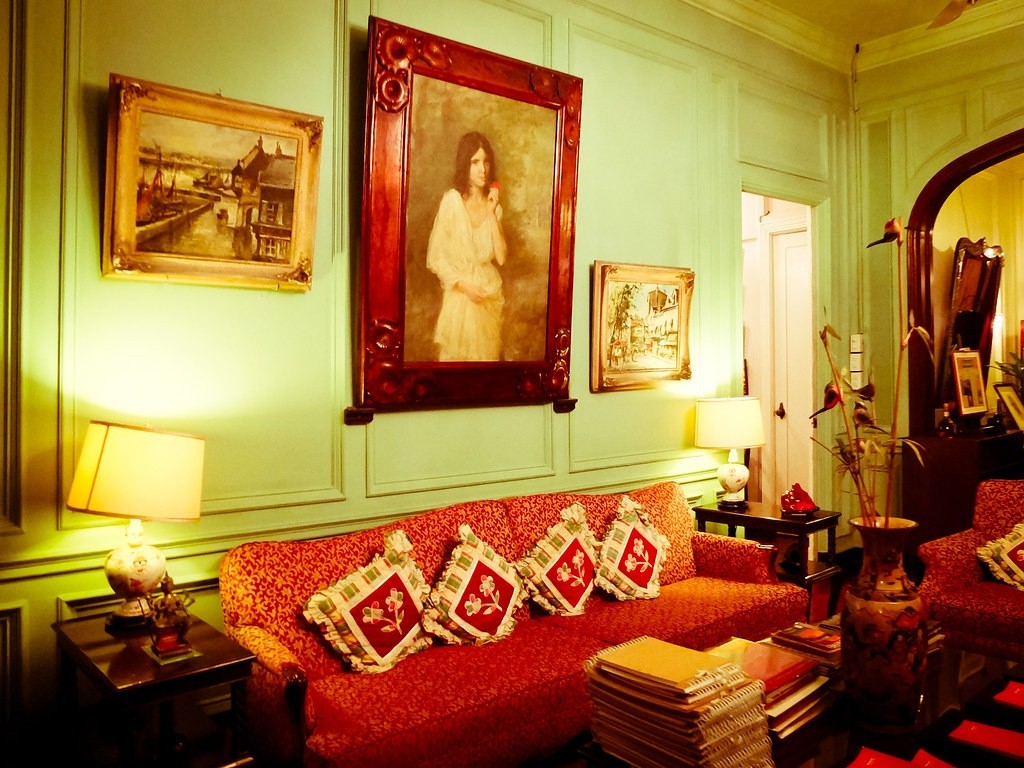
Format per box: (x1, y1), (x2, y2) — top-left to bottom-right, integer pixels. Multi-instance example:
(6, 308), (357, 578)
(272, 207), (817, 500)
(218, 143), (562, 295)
(589, 259), (695, 393)
(992, 383), (1024, 431)
(102, 72), (325, 293)
(351, 14), (584, 415)
(935, 237), (1006, 408)
(950, 347), (989, 417)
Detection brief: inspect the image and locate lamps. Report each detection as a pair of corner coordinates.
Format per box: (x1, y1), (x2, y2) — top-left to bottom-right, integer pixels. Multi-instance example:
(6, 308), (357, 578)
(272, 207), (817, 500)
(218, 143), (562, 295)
(66, 419), (206, 633)
(695, 396), (767, 509)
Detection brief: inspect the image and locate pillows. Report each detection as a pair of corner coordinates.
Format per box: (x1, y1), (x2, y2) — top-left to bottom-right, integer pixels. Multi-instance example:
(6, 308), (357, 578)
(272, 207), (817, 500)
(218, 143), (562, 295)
(421, 522), (530, 645)
(594, 495), (671, 600)
(976, 523), (1024, 591)
(303, 530), (432, 674)
(517, 502), (603, 616)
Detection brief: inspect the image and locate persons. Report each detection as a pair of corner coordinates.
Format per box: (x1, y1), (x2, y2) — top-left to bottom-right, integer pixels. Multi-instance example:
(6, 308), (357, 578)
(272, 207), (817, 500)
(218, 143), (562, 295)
(152, 569), (182, 620)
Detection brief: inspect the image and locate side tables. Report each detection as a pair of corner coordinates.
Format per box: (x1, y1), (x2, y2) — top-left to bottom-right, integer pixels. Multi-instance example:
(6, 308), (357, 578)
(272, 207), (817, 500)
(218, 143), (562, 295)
(51, 610), (257, 768)
(692, 501), (844, 623)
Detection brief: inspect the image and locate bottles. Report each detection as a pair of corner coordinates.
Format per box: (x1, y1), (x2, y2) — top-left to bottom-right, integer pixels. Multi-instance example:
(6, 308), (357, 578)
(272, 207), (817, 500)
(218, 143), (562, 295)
(937, 402), (959, 441)
(994, 398), (1007, 435)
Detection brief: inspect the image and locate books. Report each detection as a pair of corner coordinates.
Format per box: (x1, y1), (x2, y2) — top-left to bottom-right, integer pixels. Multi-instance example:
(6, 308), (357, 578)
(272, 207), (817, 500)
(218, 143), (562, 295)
(581, 635), (776, 768)
(819, 609), (945, 654)
(758, 622), (841, 676)
(704, 638), (839, 740)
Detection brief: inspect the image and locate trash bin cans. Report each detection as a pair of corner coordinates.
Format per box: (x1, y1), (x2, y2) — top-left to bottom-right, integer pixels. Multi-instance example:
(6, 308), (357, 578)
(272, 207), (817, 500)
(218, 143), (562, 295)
(818, 547), (926, 618)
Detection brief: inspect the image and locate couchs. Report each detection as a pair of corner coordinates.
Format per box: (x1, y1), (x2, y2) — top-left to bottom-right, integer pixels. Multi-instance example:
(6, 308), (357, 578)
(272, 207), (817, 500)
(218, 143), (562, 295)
(221, 480), (810, 768)
(919, 479), (1024, 717)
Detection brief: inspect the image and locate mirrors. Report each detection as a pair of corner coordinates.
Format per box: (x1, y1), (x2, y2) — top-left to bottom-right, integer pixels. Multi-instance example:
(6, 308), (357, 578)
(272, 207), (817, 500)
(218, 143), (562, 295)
(905, 126), (1024, 435)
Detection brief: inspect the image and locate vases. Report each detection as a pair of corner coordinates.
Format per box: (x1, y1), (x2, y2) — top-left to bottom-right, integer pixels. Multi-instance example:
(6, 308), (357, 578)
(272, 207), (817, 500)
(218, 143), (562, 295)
(841, 517), (926, 736)
